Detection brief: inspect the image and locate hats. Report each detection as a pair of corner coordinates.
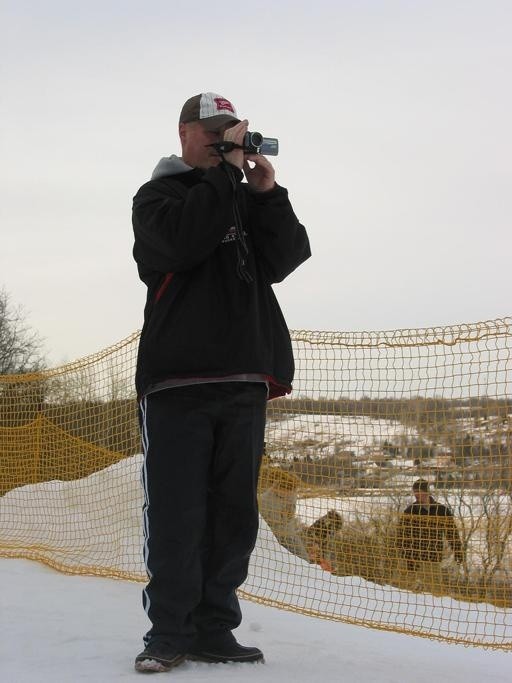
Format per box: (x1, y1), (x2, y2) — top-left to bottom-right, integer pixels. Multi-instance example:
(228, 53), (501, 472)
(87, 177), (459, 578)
(413, 478), (431, 492)
(180, 92), (241, 130)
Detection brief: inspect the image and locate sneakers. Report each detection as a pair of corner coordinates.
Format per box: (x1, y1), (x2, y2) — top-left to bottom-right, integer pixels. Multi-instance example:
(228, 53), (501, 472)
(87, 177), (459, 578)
(187, 630), (264, 662)
(135, 630), (186, 672)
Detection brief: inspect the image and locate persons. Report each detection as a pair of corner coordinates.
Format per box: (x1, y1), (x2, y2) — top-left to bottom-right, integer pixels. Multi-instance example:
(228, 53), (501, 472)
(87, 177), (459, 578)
(131, 90), (312, 675)
(261, 471), (345, 574)
(395, 478), (466, 571)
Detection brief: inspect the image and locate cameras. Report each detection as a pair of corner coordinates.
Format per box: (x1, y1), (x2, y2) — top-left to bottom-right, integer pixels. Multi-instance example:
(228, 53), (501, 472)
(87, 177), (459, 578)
(243, 131), (278, 156)
